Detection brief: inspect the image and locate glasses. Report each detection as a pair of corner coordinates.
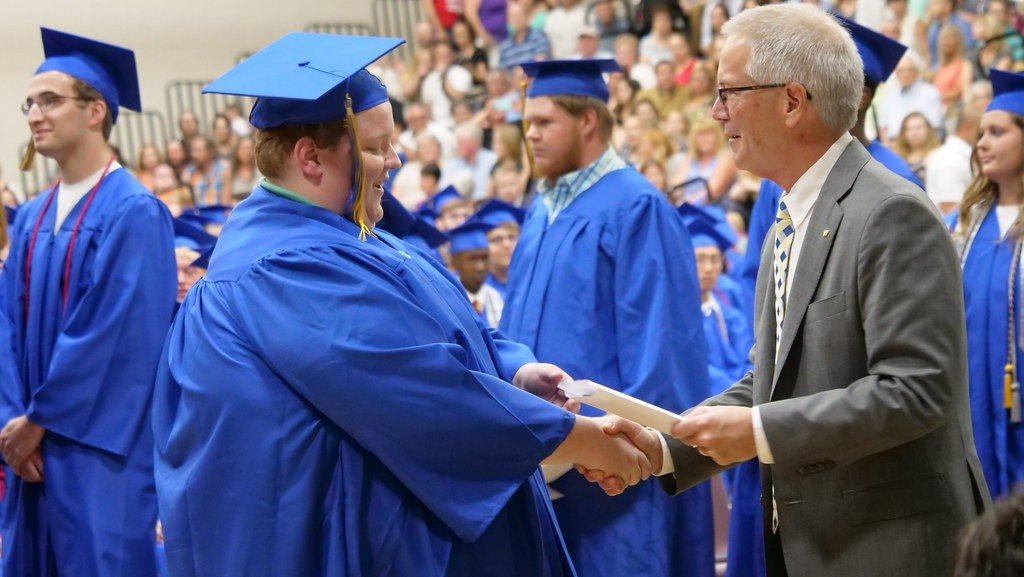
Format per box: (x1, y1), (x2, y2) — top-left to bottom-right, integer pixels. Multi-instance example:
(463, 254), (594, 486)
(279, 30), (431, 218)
(21, 94), (95, 115)
(717, 83), (812, 105)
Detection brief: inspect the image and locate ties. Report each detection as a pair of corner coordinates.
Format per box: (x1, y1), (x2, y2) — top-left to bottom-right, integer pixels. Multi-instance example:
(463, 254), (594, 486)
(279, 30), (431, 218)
(770, 202), (795, 537)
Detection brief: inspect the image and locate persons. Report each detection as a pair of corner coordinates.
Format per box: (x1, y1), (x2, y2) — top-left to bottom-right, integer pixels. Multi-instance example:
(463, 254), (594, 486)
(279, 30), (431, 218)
(1, 0), (1024, 577)
(572, 2), (992, 577)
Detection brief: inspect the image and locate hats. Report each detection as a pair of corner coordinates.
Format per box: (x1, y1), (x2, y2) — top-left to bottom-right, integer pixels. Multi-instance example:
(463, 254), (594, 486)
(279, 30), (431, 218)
(411, 184), (462, 227)
(676, 201), (736, 253)
(467, 199), (526, 232)
(831, 13), (907, 82)
(18, 27), (142, 171)
(519, 58), (624, 176)
(173, 204), (231, 269)
(445, 221), (496, 255)
(986, 68), (1024, 116)
(201, 31), (406, 242)
